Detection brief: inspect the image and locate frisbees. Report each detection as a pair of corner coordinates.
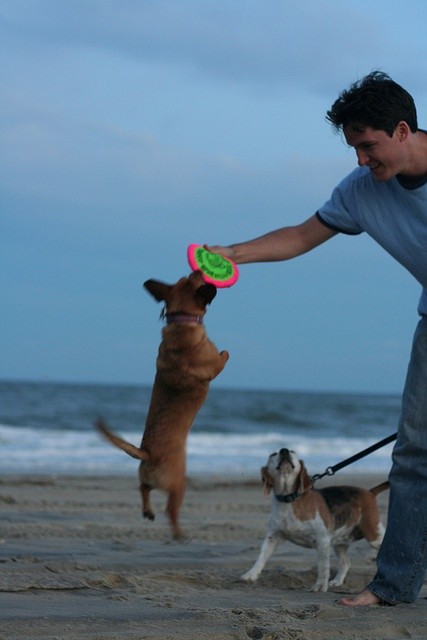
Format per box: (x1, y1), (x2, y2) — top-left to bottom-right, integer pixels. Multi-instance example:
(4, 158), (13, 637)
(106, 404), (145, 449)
(187, 244), (239, 288)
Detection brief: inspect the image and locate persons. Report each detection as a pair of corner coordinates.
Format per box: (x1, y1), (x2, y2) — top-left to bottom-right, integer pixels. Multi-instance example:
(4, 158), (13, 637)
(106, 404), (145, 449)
(201, 68), (426, 610)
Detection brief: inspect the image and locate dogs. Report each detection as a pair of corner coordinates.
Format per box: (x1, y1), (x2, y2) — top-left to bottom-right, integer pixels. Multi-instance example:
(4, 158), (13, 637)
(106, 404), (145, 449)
(93, 268), (232, 544)
(239, 447), (391, 595)
